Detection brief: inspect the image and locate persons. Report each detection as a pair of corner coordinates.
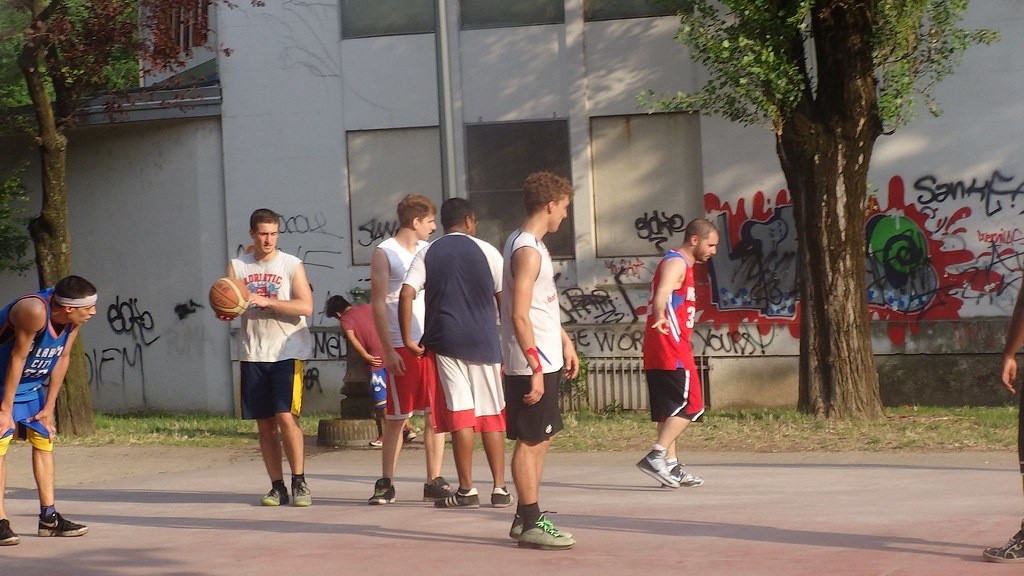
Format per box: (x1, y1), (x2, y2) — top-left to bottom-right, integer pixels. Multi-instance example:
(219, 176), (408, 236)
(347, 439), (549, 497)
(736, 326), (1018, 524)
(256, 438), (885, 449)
(326, 295), (416, 447)
(368, 193), (457, 505)
(982, 276), (1024, 564)
(0, 275), (98, 546)
(214, 209), (313, 507)
(500, 171), (579, 550)
(398, 197), (514, 508)
(636, 218), (720, 488)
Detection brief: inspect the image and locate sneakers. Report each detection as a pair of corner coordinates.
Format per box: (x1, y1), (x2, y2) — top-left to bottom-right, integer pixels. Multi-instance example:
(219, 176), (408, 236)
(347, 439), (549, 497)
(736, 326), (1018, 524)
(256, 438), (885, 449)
(661, 460), (705, 488)
(982, 529), (1024, 564)
(423, 476), (457, 502)
(38, 511), (88, 537)
(434, 488), (479, 508)
(368, 478), (395, 505)
(0, 519), (20, 546)
(491, 488), (515, 507)
(635, 449), (681, 488)
(403, 426), (416, 444)
(517, 516), (575, 550)
(292, 482), (311, 506)
(368, 435), (384, 446)
(260, 486), (289, 506)
(509, 511), (572, 539)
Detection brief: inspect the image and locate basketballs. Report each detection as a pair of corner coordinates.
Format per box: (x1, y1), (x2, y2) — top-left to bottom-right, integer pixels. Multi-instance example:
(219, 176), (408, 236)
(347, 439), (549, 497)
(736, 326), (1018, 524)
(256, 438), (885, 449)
(208, 275), (250, 319)
